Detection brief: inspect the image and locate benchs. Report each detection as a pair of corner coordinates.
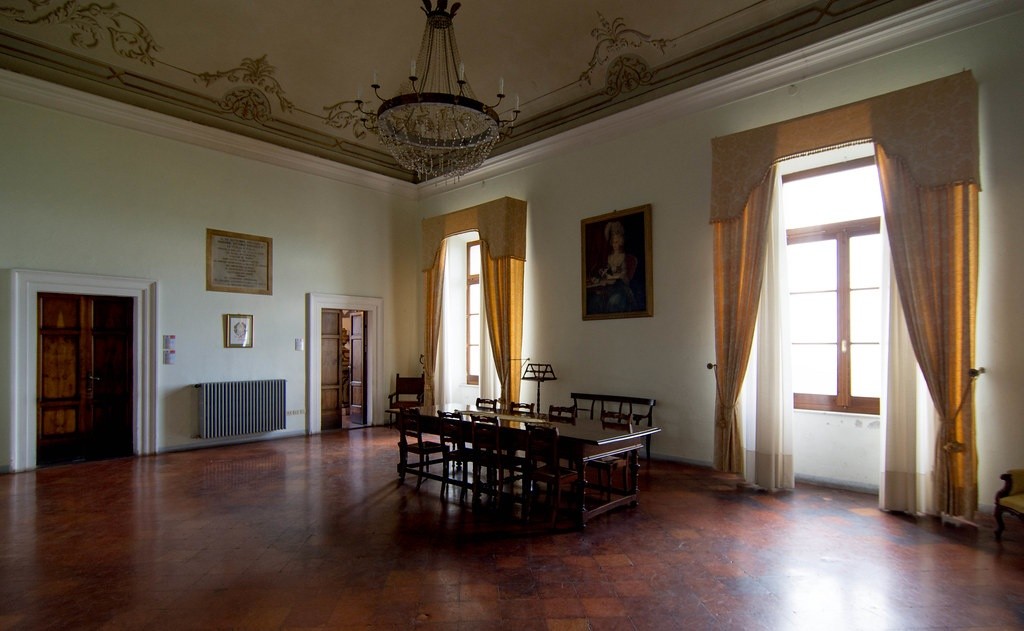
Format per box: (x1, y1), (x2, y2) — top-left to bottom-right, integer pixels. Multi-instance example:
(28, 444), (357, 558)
(570, 393), (657, 462)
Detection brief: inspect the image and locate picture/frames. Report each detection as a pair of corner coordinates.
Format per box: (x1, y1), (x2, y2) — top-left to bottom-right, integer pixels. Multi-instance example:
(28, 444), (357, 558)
(225, 314), (253, 348)
(580, 204), (654, 320)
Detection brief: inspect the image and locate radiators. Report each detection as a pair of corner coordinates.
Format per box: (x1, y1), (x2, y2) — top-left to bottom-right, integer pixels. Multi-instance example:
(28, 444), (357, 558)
(194, 379), (287, 439)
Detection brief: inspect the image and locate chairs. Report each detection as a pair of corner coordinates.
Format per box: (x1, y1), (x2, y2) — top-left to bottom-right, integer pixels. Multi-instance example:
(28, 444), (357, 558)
(994, 469), (1024, 540)
(387, 373), (634, 532)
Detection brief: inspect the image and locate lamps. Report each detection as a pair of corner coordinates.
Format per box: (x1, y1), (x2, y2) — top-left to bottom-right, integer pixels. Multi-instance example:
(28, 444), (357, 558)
(355, 0), (521, 187)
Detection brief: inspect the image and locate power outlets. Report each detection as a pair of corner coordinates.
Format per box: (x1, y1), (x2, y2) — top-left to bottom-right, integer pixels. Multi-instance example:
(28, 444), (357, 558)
(288, 409), (307, 416)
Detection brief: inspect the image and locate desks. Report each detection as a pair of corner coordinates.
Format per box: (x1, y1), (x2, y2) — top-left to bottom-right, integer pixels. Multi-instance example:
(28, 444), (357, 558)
(386, 405), (661, 533)
